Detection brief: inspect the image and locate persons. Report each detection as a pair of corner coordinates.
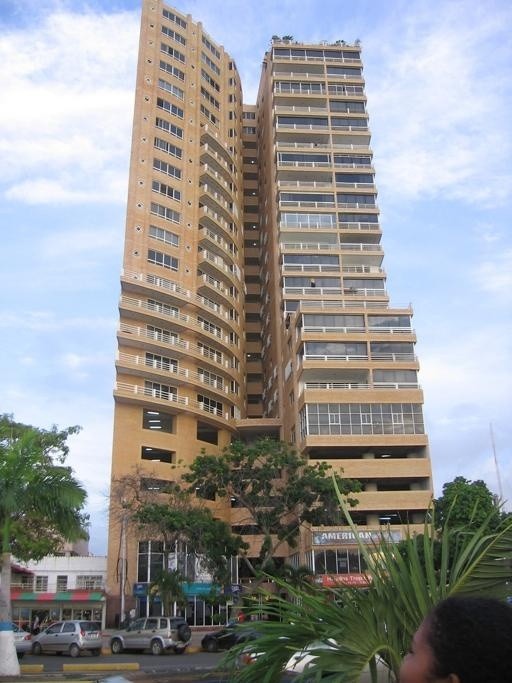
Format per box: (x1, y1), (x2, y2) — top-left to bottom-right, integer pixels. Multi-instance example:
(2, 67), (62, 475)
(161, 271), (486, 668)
(31, 613), (40, 634)
(395, 593), (511, 681)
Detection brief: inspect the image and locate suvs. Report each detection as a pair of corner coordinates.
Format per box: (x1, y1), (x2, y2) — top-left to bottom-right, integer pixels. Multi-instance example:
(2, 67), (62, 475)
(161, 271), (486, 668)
(110, 616), (192, 656)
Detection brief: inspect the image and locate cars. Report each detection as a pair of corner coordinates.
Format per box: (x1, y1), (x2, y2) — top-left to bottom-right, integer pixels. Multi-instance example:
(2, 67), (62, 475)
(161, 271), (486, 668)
(12, 621), (33, 659)
(201, 620), (262, 654)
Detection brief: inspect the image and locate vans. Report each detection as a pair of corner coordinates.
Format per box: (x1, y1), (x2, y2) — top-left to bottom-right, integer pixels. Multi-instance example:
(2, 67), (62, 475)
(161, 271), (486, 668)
(30, 619), (104, 658)
